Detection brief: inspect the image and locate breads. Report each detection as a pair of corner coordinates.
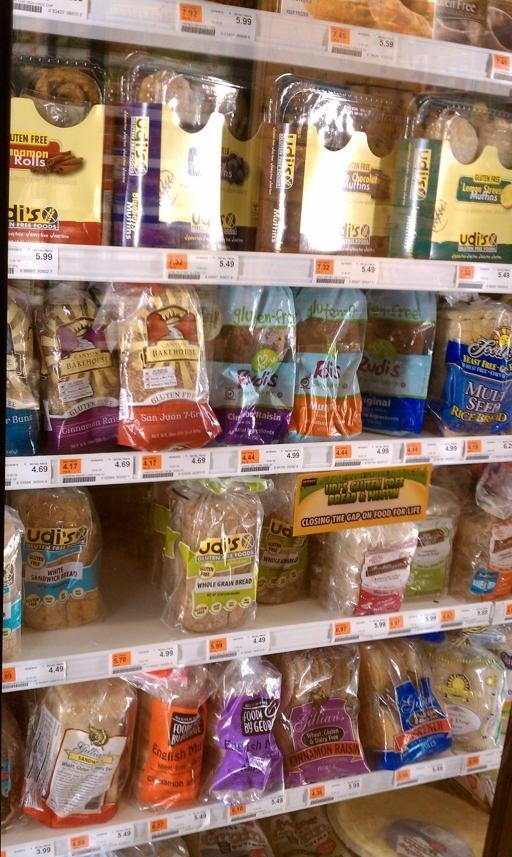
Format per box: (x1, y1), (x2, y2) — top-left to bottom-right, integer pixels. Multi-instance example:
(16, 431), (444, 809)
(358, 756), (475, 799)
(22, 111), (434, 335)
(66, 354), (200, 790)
(8, 29), (512, 263)
(3, 461), (511, 663)
(4, 623), (512, 829)
(116, 769), (498, 856)
(6, 279), (512, 455)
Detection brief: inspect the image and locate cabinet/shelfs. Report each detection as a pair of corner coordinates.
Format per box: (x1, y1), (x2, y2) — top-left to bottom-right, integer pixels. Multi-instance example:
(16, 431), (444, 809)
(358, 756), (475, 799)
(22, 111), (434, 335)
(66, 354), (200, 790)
(0, 0), (511, 857)
